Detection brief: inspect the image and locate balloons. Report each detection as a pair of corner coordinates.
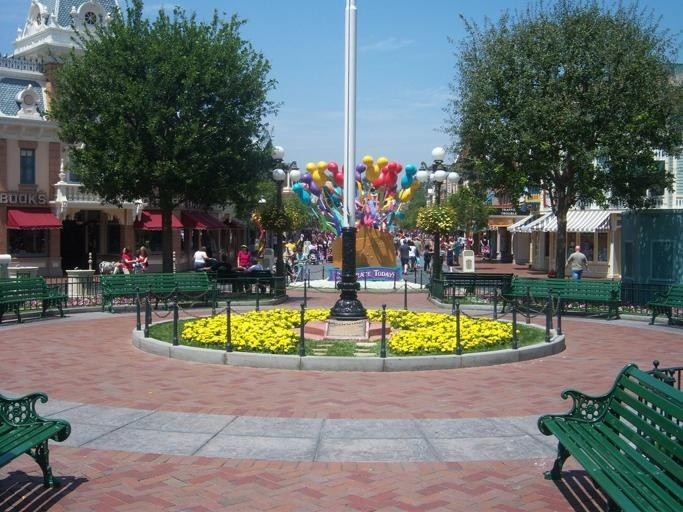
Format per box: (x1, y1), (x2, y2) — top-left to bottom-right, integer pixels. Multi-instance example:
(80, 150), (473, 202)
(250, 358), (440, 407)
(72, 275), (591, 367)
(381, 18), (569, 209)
(290, 155), (421, 236)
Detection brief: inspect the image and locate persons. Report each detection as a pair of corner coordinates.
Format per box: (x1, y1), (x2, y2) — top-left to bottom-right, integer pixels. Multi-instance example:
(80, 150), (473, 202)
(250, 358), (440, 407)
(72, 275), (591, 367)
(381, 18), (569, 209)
(198, 252), (244, 293)
(391, 228), (490, 276)
(236, 243), (253, 269)
(111, 245), (150, 284)
(564, 245), (588, 281)
(241, 256), (266, 294)
(253, 230), (336, 283)
(192, 245), (219, 272)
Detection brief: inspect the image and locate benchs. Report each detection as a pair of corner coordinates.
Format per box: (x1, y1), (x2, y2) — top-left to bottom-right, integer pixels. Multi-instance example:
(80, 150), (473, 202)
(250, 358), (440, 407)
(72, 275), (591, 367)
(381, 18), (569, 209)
(0, 393), (70, 487)
(0, 270), (273, 324)
(443, 272), (682, 325)
(537, 362), (682, 511)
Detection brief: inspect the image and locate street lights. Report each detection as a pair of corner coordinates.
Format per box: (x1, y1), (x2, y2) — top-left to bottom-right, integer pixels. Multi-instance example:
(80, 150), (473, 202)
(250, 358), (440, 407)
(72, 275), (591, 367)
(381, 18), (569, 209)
(271, 144), (300, 275)
(414, 147), (460, 279)
